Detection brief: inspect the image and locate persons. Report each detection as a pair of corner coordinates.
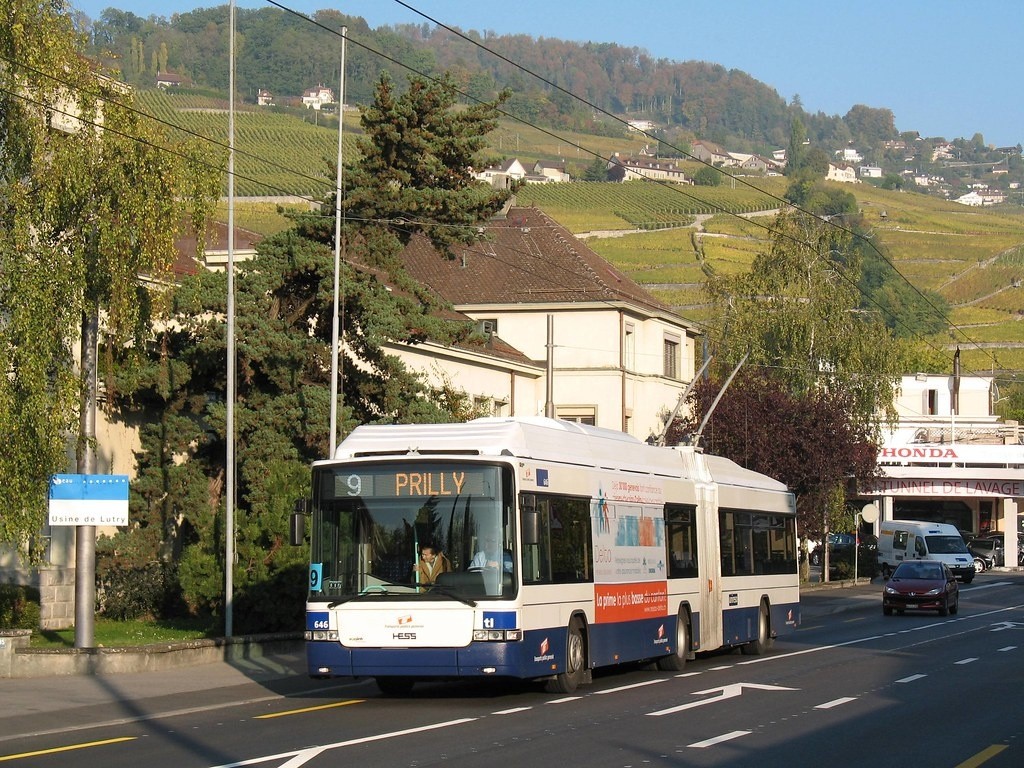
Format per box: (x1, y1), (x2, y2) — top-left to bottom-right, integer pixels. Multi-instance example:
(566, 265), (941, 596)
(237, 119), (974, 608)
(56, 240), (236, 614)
(982, 518), (991, 532)
(929, 569), (942, 578)
(411, 544), (452, 593)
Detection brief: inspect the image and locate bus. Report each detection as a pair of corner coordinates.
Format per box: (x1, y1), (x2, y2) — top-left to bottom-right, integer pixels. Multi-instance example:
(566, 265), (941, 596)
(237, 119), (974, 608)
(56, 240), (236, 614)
(290, 411), (801, 698)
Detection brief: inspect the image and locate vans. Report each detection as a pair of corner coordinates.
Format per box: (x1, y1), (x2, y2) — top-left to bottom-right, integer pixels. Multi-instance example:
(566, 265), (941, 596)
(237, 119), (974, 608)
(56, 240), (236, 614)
(878, 520), (977, 584)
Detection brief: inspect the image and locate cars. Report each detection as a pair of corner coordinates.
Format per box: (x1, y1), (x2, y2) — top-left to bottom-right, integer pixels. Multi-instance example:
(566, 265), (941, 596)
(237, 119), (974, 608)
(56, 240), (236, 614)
(958, 530), (1024, 572)
(812, 532), (879, 566)
(882, 560), (958, 617)
